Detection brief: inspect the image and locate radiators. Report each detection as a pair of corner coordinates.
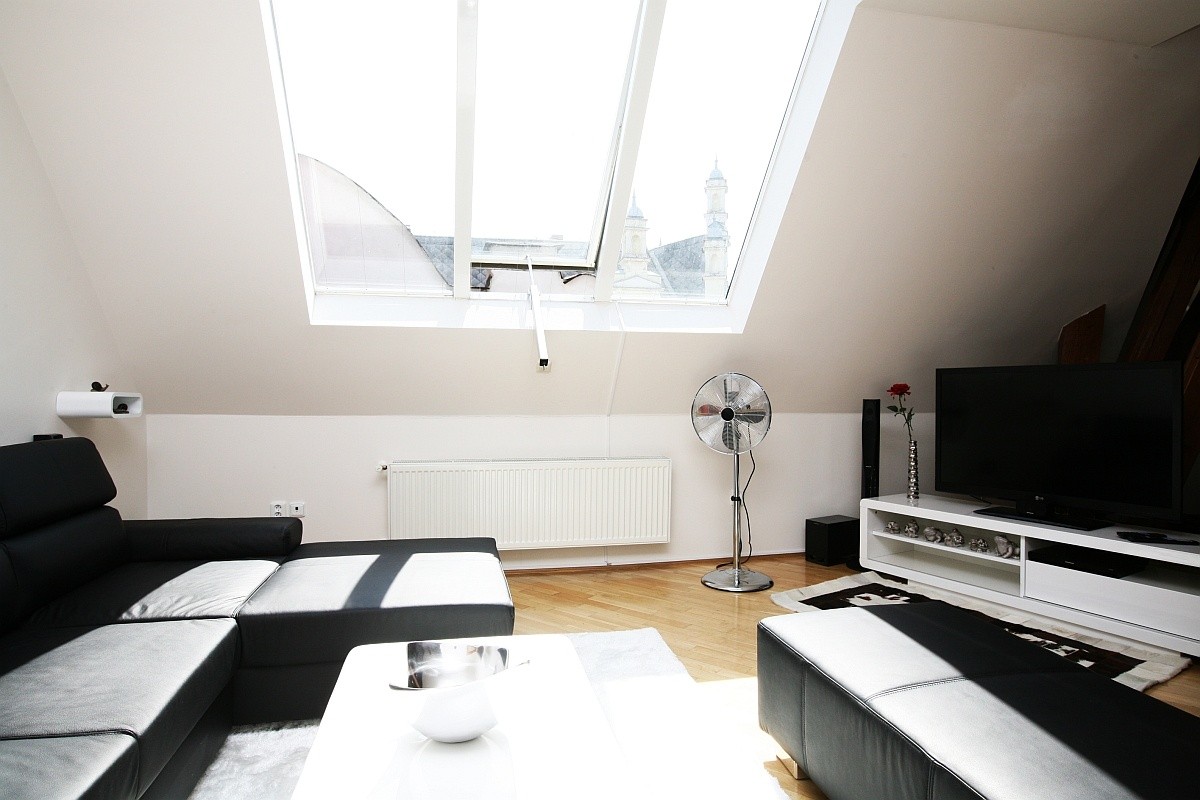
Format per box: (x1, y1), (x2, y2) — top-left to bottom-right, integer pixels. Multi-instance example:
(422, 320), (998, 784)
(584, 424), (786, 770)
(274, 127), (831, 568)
(374, 456), (674, 551)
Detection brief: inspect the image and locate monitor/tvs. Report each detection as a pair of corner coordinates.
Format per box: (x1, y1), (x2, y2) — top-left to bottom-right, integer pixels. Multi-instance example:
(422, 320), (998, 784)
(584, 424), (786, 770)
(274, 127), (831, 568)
(935, 360), (1184, 531)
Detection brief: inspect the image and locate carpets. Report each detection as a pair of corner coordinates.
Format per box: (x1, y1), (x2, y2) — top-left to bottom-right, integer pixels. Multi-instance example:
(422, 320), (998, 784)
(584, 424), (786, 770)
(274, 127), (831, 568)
(768, 569), (1193, 693)
(185, 674), (823, 799)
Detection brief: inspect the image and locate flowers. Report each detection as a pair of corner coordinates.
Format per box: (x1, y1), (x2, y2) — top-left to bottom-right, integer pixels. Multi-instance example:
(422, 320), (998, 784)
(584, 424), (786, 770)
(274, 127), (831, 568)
(886, 383), (915, 441)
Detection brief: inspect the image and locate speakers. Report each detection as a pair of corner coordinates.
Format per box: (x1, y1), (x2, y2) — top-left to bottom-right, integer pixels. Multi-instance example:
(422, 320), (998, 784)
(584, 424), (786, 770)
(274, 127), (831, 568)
(805, 514), (860, 566)
(860, 398), (881, 501)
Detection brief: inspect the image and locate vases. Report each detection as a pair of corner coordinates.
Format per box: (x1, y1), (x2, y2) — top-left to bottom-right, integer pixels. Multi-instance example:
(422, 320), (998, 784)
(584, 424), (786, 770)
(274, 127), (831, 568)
(907, 440), (920, 499)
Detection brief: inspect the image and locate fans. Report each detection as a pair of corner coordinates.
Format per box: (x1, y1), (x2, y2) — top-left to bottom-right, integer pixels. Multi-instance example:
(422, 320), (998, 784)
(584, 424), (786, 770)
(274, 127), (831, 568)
(690, 373), (775, 593)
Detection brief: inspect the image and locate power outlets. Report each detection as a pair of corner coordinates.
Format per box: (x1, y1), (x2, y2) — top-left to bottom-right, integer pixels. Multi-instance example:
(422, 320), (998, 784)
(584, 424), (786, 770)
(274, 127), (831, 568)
(270, 502), (287, 517)
(288, 500), (305, 518)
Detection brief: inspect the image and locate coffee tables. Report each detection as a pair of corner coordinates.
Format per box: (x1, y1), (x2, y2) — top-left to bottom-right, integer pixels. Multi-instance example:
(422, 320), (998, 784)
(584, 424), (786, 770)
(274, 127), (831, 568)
(288, 625), (788, 800)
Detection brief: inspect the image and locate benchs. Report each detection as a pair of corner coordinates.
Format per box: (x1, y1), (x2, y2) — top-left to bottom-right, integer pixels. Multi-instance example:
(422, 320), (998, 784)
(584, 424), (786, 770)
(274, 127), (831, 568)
(753, 600), (1200, 800)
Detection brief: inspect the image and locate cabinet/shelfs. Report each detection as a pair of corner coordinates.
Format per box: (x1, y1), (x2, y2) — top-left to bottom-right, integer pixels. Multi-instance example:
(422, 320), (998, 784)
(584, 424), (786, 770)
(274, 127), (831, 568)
(858, 492), (1200, 659)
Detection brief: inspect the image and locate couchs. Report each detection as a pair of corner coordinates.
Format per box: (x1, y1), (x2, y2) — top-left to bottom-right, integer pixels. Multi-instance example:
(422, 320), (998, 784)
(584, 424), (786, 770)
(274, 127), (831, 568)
(0, 435), (514, 800)
